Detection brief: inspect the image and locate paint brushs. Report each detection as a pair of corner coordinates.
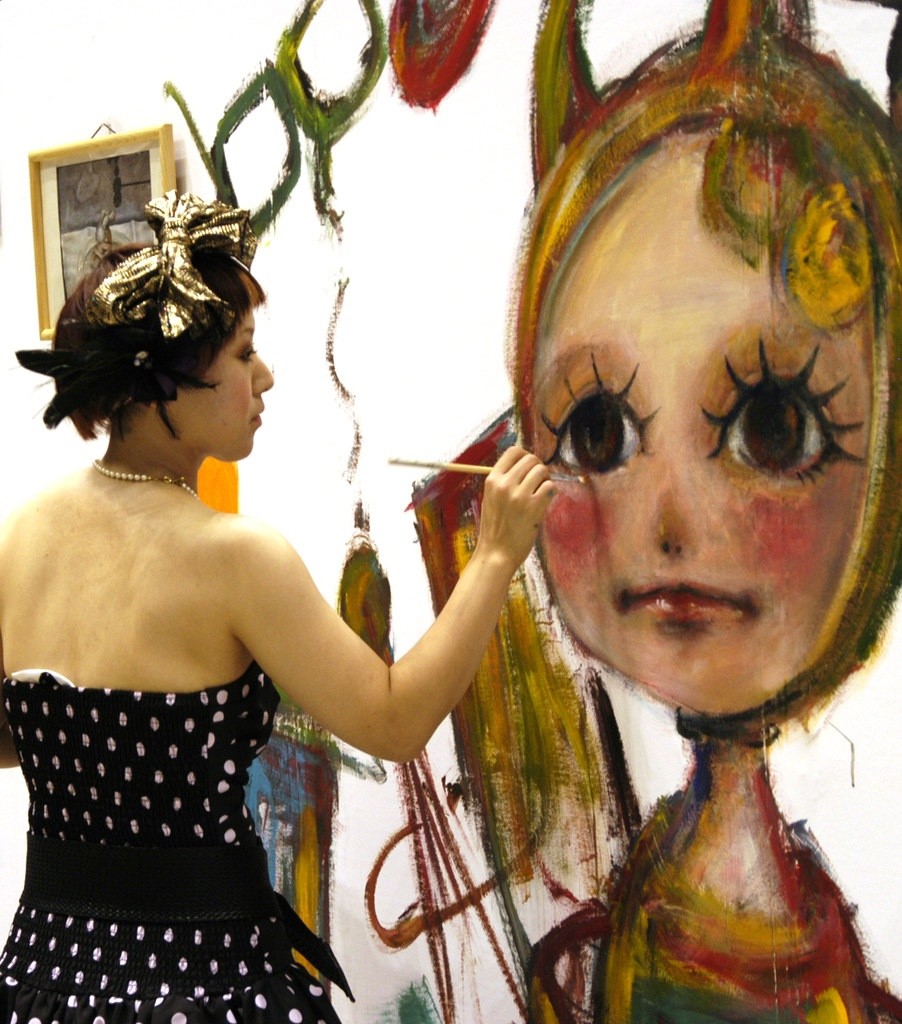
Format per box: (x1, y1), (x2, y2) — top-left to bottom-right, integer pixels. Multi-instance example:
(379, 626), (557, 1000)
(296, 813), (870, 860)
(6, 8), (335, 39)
(389, 456), (586, 484)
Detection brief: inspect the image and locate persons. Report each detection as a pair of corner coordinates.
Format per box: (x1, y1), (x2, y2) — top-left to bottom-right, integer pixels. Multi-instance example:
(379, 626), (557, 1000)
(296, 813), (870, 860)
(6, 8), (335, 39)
(0, 186), (553, 1024)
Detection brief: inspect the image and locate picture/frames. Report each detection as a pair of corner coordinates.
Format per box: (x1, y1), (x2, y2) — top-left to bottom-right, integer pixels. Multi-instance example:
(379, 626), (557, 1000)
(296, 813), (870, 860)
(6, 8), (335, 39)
(27, 122), (179, 340)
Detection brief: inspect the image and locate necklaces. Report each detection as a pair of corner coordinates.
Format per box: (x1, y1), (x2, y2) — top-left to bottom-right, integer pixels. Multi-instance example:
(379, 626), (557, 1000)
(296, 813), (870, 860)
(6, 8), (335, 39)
(93, 457), (202, 498)
